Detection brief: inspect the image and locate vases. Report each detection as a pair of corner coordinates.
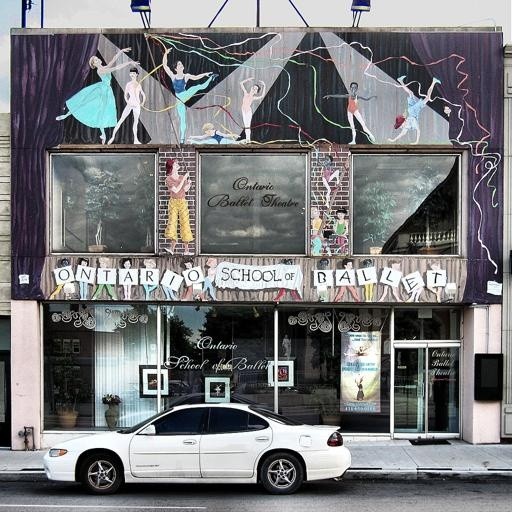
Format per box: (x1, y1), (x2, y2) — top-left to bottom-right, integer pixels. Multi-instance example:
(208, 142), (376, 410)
(57, 410), (78, 427)
(105, 402), (119, 429)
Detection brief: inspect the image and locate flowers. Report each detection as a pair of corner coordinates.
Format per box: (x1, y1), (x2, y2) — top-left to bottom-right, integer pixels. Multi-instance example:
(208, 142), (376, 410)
(102, 393), (121, 405)
(57, 388), (79, 411)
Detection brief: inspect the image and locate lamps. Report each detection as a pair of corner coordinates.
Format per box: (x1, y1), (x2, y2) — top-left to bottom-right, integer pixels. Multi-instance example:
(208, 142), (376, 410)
(192, 283), (202, 302)
(130, 0), (152, 29)
(350, 0), (370, 27)
(317, 284), (327, 302)
(444, 283), (456, 303)
(63, 282), (75, 300)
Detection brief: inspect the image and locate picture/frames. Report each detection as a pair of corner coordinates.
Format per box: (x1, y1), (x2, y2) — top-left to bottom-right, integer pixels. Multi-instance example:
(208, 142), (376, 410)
(139, 364), (172, 398)
(351, 154), (462, 257)
(268, 360), (294, 387)
(204, 376), (231, 404)
(198, 152), (308, 257)
(49, 152), (158, 256)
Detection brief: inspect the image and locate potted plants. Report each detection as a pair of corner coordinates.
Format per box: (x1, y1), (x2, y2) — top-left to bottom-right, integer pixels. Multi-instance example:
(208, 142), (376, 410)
(83, 170), (123, 252)
(357, 176), (398, 254)
(127, 162), (154, 253)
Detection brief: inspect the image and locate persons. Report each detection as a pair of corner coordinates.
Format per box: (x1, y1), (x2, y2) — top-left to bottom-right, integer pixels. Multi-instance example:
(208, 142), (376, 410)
(107, 68), (146, 145)
(239, 77), (266, 142)
(187, 123), (246, 145)
(323, 82), (377, 145)
(162, 48), (219, 143)
(278, 368), (288, 380)
(309, 155), (349, 253)
(189, 373), (203, 403)
(283, 334), (291, 357)
(151, 378), (157, 389)
(276, 258), (444, 303)
(388, 76), (441, 146)
(48, 258), (222, 301)
(66, 47), (141, 146)
(48, 378), (82, 415)
(231, 383), (236, 395)
(163, 160), (195, 255)
(214, 385), (222, 396)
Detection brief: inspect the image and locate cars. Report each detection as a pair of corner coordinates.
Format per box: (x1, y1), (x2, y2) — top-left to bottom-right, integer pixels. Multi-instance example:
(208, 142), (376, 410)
(287, 386), (312, 394)
(166, 379), (190, 396)
(119, 391), (326, 429)
(44, 402), (352, 495)
